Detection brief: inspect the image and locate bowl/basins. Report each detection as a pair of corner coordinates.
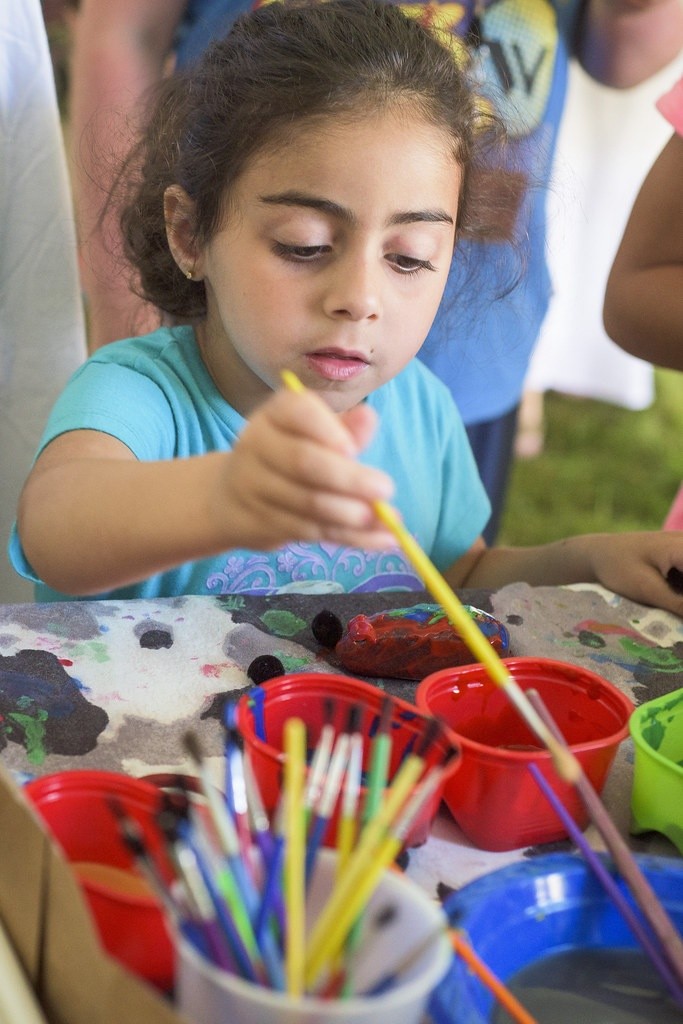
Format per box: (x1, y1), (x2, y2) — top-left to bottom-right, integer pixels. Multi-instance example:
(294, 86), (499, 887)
(433, 855), (682, 1023)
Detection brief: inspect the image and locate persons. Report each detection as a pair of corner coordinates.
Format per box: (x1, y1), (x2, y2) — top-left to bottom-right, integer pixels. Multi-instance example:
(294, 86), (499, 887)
(72, 1), (682, 554)
(8, 5), (683, 623)
(601, 83), (683, 543)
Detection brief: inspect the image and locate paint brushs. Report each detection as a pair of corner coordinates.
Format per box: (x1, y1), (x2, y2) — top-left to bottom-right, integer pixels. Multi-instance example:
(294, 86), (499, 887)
(275, 363), (582, 791)
(95, 689), (464, 1004)
(390, 687), (683, 1024)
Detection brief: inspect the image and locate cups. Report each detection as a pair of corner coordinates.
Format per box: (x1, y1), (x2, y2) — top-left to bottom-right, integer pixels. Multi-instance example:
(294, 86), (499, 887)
(18, 768), (237, 989)
(418, 657), (638, 854)
(236, 675), (459, 851)
(628, 687), (681, 858)
(164, 850), (455, 1022)
